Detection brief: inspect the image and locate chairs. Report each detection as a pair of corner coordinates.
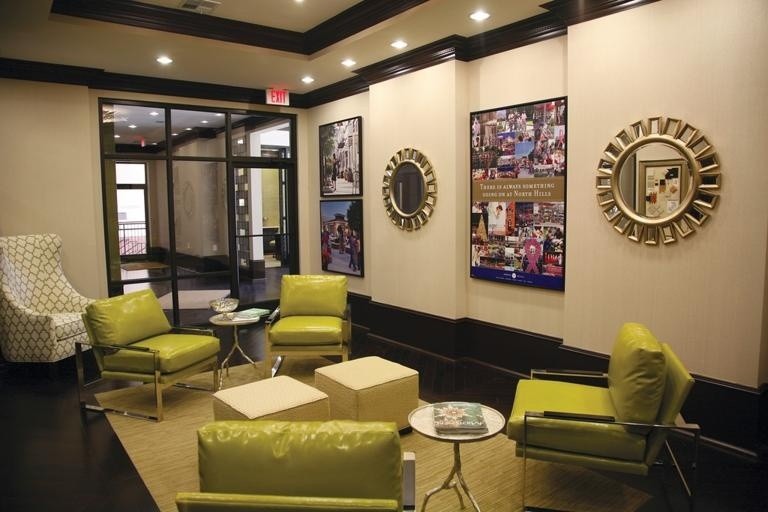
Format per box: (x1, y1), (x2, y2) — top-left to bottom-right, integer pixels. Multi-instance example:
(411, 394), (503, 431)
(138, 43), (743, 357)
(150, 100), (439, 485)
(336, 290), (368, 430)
(75, 313), (220, 422)
(264, 302), (351, 377)
(507, 341), (700, 512)
(176, 487), (403, 512)
(0, 233), (96, 383)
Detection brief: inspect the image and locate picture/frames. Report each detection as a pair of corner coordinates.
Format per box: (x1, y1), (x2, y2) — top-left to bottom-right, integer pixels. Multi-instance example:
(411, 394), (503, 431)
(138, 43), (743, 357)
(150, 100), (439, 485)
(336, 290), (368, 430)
(639, 159), (689, 218)
(320, 199), (364, 276)
(318, 116), (362, 196)
(470, 96), (567, 291)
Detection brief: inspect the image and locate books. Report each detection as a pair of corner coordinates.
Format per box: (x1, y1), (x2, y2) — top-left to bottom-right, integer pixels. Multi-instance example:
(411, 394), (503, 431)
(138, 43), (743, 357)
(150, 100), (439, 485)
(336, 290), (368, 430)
(433, 402), (489, 434)
(232, 307), (270, 323)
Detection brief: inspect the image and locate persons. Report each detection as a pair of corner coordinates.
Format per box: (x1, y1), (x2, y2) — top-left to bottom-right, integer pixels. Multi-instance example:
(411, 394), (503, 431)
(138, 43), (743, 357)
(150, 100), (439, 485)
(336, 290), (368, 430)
(473, 103), (564, 274)
(322, 224), (361, 271)
(331, 153), (338, 190)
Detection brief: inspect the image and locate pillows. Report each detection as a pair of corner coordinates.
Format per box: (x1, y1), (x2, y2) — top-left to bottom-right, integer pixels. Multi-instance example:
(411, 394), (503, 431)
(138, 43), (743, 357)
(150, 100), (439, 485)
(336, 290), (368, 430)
(608, 322), (666, 435)
(197, 420), (402, 501)
(85, 289), (173, 352)
(279, 276), (348, 318)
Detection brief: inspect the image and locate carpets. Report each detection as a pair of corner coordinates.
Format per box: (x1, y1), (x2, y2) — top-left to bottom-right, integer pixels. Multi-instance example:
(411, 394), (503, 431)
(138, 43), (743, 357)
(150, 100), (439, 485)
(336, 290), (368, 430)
(156, 290), (231, 310)
(121, 261), (170, 271)
(94, 356), (653, 512)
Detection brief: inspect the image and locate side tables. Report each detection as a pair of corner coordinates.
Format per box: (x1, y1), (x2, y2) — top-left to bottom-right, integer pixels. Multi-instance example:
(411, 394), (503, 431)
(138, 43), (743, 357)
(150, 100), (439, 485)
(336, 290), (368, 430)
(409, 402), (507, 512)
(208, 312), (261, 390)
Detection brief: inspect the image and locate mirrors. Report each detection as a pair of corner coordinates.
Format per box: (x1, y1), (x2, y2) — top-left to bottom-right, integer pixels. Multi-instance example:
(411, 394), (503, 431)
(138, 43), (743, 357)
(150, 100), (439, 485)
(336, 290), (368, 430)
(383, 148), (437, 231)
(596, 118), (721, 245)
